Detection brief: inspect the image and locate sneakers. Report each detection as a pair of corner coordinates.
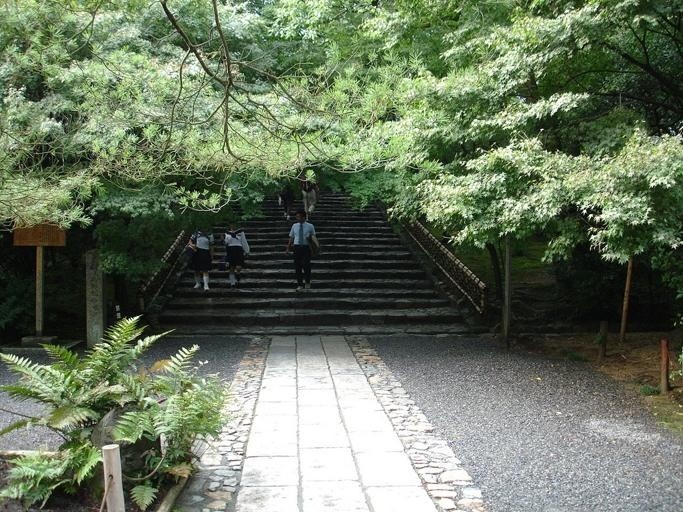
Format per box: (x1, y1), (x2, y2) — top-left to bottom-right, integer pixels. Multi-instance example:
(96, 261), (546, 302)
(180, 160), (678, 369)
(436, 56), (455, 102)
(308, 210), (312, 219)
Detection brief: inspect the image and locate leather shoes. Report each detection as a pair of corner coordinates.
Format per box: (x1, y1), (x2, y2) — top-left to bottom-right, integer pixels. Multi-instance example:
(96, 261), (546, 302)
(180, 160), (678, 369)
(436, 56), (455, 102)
(296, 284), (311, 292)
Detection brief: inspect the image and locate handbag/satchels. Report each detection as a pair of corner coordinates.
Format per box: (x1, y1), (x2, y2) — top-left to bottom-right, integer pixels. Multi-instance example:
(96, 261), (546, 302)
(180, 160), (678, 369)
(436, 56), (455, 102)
(186, 244), (198, 258)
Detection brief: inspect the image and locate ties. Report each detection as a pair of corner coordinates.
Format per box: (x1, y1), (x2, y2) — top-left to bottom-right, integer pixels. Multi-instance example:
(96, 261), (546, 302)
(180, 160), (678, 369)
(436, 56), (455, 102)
(299, 223), (304, 244)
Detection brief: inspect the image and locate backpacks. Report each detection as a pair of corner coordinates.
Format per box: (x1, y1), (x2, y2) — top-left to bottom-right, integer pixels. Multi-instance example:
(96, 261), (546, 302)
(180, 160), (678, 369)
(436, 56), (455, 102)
(303, 182), (312, 192)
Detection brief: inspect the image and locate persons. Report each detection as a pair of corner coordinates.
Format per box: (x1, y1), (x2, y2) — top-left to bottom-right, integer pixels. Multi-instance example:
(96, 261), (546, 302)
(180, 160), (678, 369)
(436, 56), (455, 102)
(299, 175), (319, 222)
(223, 225), (250, 286)
(286, 209), (321, 291)
(187, 224), (215, 291)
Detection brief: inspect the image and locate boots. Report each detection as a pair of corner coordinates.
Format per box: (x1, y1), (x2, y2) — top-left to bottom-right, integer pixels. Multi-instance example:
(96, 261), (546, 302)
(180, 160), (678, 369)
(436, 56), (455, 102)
(193, 275), (210, 291)
(230, 273), (241, 289)
